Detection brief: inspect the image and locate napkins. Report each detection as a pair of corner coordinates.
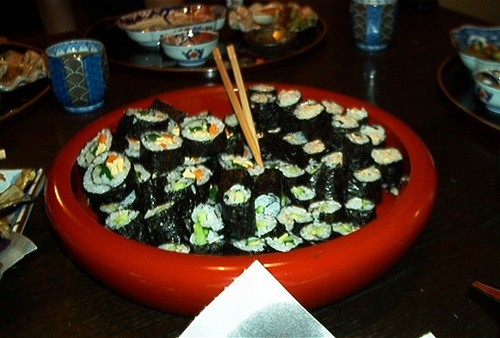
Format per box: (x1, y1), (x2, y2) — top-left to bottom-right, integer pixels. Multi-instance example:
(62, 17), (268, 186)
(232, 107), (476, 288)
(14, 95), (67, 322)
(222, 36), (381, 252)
(178, 260), (334, 338)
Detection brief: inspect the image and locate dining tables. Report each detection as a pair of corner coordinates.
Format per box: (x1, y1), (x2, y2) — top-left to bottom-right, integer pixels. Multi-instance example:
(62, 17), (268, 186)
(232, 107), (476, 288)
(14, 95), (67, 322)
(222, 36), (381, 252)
(0, 0), (500, 338)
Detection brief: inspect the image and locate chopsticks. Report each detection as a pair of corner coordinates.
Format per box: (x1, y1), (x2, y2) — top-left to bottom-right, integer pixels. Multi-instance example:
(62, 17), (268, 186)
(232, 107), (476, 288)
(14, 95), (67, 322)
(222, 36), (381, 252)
(211, 44), (264, 171)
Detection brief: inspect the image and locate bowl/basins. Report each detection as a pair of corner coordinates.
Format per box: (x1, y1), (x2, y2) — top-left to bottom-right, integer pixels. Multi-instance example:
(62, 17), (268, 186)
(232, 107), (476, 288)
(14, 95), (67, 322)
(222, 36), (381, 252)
(160, 29), (220, 66)
(245, 27), (297, 55)
(448, 24), (500, 72)
(116, 3), (227, 53)
(471, 67), (500, 114)
(44, 82), (439, 318)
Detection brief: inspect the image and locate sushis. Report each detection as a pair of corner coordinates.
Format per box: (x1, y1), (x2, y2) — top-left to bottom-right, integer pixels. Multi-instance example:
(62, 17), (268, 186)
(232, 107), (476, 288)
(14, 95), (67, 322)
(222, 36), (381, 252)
(75, 86), (404, 255)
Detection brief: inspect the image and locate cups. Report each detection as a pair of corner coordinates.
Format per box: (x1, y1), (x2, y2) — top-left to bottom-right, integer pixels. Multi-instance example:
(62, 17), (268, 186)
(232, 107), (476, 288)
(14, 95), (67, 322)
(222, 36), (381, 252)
(349, 0), (397, 54)
(43, 38), (109, 115)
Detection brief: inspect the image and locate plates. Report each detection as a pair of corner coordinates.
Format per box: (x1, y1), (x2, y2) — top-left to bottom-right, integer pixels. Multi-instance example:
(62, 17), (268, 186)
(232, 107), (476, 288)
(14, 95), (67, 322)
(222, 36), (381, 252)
(0, 39), (51, 122)
(0, 162), (45, 236)
